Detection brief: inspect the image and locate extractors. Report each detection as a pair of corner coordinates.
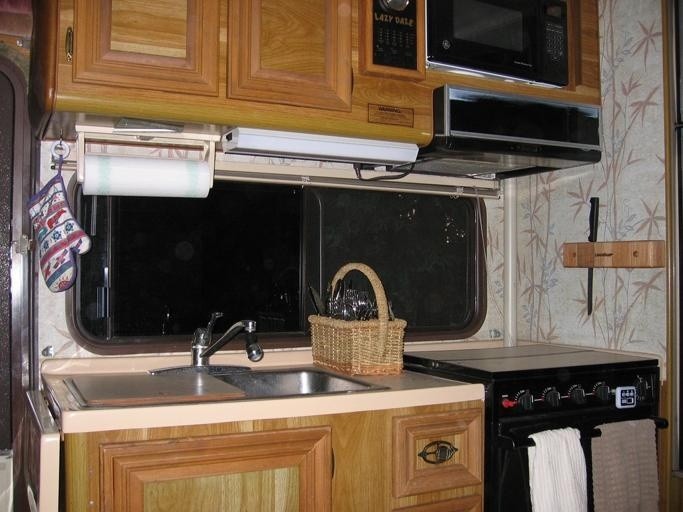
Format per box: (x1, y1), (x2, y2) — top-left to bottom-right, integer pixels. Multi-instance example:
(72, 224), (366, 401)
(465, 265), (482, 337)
(388, 84), (605, 185)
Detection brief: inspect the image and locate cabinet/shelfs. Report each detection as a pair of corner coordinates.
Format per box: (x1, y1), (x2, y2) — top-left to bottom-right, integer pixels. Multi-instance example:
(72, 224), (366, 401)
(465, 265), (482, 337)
(34, 1), (360, 139)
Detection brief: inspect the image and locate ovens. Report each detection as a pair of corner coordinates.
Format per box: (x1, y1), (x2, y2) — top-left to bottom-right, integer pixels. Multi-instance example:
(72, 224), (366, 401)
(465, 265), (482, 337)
(497, 420), (665, 512)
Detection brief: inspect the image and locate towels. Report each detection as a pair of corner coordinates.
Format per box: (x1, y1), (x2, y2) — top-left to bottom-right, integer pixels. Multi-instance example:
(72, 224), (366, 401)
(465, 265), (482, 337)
(527, 417), (659, 512)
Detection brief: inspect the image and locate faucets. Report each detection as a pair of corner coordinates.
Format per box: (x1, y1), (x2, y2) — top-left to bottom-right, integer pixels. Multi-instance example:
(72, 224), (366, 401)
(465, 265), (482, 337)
(191, 306), (264, 366)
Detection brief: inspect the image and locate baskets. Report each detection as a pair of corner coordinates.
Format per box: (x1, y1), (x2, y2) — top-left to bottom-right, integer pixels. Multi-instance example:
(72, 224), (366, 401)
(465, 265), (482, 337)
(308, 262), (408, 377)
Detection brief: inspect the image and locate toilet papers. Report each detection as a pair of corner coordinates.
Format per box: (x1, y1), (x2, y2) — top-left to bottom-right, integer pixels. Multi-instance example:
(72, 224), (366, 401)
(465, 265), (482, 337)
(81, 155), (211, 198)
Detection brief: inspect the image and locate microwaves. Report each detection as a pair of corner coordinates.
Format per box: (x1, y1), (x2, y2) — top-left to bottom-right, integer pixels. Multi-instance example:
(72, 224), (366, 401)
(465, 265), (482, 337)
(420, 1), (599, 94)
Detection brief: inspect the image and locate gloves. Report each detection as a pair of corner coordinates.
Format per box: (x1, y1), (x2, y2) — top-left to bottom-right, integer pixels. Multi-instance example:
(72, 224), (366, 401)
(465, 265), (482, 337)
(26, 155), (92, 293)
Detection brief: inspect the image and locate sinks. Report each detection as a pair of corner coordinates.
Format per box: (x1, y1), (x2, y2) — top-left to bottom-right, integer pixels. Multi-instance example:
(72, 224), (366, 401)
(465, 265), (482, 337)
(169, 366), (390, 403)
